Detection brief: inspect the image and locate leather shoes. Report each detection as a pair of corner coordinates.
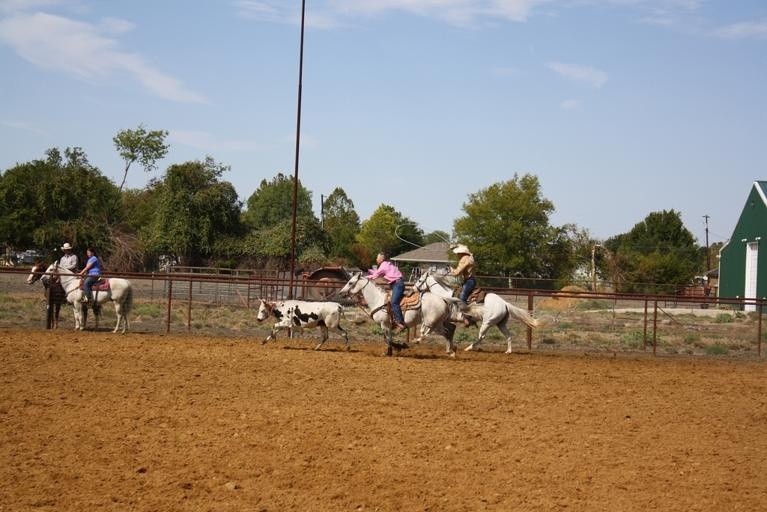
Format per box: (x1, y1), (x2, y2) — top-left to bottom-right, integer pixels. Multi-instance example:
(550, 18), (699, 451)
(396, 323), (407, 335)
(464, 318), (472, 327)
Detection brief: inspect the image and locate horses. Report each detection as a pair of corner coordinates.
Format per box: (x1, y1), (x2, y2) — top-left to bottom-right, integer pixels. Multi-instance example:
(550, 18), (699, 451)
(40, 259), (133, 335)
(26, 261), (102, 330)
(338, 271), (490, 356)
(411, 264), (552, 355)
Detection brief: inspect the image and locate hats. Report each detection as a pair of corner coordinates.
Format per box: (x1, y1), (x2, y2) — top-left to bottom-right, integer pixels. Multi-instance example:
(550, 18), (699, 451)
(61, 243), (72, 252)
(452, 246), (471, 256)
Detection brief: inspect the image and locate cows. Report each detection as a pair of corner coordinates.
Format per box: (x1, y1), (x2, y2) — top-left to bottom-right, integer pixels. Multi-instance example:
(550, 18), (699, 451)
(254, 297), (361, 352)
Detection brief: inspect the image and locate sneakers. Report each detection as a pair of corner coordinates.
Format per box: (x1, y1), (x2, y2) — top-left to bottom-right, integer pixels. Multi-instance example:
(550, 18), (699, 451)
(80, 298), (92, 302)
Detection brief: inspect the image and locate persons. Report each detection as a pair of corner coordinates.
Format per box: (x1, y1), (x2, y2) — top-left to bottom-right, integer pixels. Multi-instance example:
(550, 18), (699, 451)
(448, 246), (476, 327)
(77, 248), (103, 303)
(365, 252), (406, 329)
(59, 242), (79, 273)
